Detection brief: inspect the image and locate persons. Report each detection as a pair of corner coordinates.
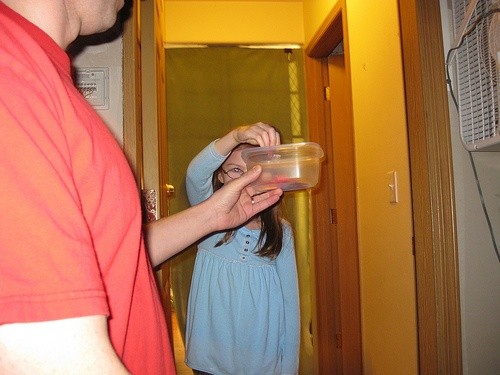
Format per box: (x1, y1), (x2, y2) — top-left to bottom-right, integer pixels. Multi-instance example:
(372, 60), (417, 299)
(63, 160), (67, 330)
(1, 1), (285, 374)
(183, 120), (301, 375)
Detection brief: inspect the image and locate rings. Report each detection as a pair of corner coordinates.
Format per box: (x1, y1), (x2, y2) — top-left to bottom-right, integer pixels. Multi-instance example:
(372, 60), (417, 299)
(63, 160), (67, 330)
(251, 197), (255, 204)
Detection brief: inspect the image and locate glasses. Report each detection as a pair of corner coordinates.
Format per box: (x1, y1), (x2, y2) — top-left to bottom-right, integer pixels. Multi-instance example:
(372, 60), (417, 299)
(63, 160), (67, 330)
(219, 165), (244, 179)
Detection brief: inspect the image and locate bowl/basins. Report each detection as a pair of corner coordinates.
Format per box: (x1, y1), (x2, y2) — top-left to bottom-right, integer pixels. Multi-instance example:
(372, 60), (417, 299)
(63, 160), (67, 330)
(241, 142), (326, 192)
